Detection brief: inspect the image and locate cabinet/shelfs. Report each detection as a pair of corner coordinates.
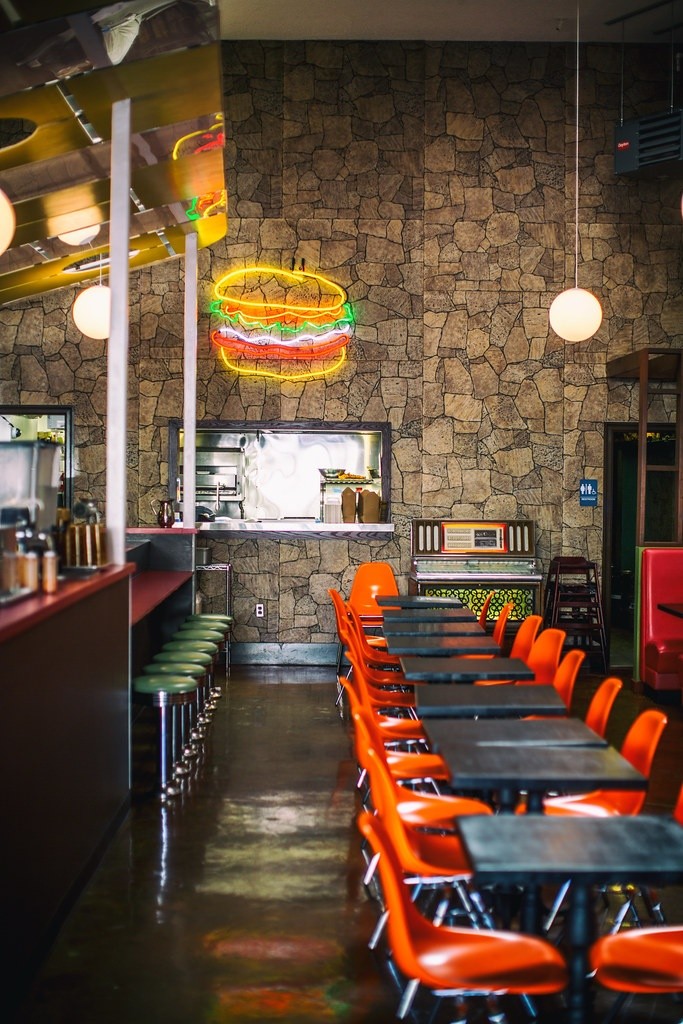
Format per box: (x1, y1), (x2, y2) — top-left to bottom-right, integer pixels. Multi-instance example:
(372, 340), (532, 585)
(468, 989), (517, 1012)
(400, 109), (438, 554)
(196, 562), (231, 668)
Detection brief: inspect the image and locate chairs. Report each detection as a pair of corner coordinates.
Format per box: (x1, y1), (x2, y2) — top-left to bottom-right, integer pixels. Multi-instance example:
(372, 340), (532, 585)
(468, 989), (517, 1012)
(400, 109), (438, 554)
(589, 925), (683, 1024)
(520, 649), (586, 721)
(542, 555), (611, 676)
(341, 615), (426, 693)
(540, 784), (683, 979)
(519, 676), (624, 795)
(516, 708), (668, 815)
(337, 562), (401, 672)
(513, 628), (566, 686)
(479, 591), (496, 630)
(335, 601), (405, 706)
(362, 742), (476, 948)
(344, 649), (429, 804)
(339, 674), (451, 848)
(474, 615), (544, 686)
(330, 589), (384, 675)
(340, 630), (419, 720)
(450, 602), (513, 659)
(356, 810), (572, 1024)
(353, 708), (495, 885)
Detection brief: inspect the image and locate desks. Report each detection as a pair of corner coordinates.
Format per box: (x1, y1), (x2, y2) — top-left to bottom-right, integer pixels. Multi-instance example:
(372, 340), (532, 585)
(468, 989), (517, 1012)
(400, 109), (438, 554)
(399, 656), (535, 719)
(414, 684), (567, 801)
(453, 814), (683, 1024)
(657, 602), (683, 619)
(382, 623), (486, 638)
(386, 635), (501, 685)
(422, 718), (609, 929)
(375, 595), (463, 610)
(382, 608), (477, 624)
(436, 742), (650, 936)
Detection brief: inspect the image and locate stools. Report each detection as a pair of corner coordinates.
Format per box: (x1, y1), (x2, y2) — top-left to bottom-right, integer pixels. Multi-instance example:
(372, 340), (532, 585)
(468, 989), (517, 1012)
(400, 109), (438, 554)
(132, 675), (199, 804)
(143, 663), (206, 793)
(186, 613), (234, 709)
(164, 641), (219, 755)
(171, 630), (225, 738)
(179, 621), (230, 724)
(155, 652), (213, 766)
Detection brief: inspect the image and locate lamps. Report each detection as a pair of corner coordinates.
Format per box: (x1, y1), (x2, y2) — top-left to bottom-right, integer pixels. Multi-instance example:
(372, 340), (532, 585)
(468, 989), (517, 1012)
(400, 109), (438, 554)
(72, 254), (111, 339)
(548, 0), (603, 343)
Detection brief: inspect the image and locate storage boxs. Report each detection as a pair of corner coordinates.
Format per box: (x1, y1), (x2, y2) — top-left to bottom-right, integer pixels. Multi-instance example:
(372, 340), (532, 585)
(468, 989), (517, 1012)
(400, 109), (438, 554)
(195, 547), (212, 565)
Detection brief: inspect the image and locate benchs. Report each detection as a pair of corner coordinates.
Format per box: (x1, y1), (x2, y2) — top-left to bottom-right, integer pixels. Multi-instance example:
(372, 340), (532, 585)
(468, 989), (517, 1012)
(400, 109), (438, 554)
(639, 548), (683, 704)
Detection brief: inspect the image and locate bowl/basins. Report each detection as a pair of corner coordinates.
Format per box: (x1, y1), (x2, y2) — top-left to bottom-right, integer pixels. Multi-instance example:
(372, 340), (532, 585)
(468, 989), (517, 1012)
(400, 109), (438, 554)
(319, 468), (345, 479)
(195, 548), (212, 565)
(369, 469), (380, 479)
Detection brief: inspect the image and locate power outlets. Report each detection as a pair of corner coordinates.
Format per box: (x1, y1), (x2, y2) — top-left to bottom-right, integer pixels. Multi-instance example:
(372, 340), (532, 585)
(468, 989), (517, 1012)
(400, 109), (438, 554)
(256, 604), (264, 617)
